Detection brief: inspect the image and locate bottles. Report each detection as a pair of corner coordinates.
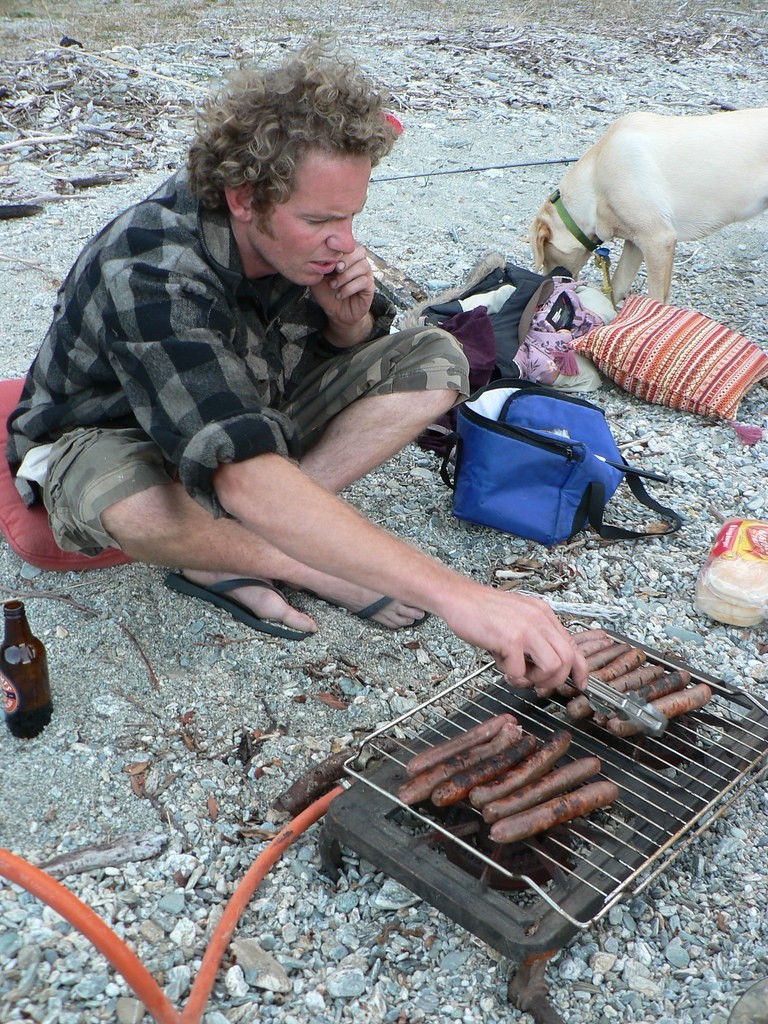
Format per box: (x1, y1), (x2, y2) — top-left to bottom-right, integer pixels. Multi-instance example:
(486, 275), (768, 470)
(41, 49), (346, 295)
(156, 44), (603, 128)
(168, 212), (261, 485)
(1, 596), (51, 745)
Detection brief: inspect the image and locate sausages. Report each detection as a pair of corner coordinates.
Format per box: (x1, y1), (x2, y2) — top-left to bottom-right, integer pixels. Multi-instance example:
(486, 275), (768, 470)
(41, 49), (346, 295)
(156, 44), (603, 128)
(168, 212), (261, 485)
(399, 626), (711, 843)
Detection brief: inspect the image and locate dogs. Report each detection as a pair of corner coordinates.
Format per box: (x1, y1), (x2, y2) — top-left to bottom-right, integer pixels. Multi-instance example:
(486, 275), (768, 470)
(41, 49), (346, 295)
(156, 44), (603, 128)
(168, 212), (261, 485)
(528, 107), (768, 305)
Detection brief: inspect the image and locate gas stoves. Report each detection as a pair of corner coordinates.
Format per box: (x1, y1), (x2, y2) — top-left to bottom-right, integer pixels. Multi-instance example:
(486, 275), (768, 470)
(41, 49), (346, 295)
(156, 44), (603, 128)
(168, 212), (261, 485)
(314, 633), (768, 1021)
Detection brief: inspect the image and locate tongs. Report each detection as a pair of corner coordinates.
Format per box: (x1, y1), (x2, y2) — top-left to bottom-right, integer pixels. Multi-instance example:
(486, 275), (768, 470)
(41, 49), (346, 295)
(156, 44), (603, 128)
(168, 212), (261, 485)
(520, 655), (669, 738)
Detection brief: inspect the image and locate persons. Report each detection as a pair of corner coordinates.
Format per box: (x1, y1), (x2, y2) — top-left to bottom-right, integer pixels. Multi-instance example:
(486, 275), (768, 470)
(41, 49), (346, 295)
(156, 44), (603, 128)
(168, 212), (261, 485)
(4, 39), (587, 697)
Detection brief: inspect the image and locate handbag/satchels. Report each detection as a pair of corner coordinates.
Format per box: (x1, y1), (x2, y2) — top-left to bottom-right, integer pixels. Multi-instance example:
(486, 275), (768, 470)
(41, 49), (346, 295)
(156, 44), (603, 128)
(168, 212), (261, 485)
(440, 378), (684, 546)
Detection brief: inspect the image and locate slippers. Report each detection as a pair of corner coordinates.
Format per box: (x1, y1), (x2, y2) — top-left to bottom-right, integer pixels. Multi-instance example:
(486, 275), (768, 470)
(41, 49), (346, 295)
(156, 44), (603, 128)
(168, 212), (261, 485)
(353, 591), (431, 631)
(165, 570), (311, 640)
